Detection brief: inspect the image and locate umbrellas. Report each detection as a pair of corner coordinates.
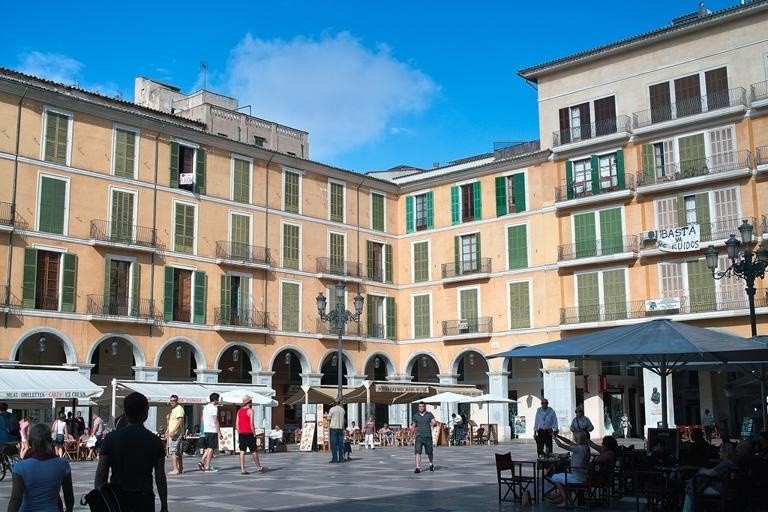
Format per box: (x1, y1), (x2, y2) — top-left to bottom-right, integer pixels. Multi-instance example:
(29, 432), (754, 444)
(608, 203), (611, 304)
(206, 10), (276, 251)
(411, 391), (475, 427)
(628, 334), (768, 434)
(206, 388), (279, 408)
(484, 318), (768, 430)
(456, 394), (520, 440)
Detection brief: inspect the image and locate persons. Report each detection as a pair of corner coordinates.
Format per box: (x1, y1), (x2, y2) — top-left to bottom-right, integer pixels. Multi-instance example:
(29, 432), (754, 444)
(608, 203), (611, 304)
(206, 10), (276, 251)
(378, 422), (394, 446)
(235, 394), (264, 474)
(452, 413), (463, 445)
(349, 421), (359, 437)
(410, 401), (438, 473)
(343, 438), (352, 461)
(533, 399), (768, 512)
(327, 400), (346, 463)
(269, 424), (301, 452)
(0, 392), (223, 512)
(363, 416), (376, 450)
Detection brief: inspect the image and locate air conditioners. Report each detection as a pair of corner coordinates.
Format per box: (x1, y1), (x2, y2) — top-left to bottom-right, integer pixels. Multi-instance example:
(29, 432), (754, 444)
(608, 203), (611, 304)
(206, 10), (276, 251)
(642, 230), (657, 240)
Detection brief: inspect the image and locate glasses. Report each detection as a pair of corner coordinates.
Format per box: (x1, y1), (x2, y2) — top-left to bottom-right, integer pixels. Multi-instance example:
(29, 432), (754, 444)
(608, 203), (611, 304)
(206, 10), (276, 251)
(170, 399), (177, 402)
(541, 402), (548, 405)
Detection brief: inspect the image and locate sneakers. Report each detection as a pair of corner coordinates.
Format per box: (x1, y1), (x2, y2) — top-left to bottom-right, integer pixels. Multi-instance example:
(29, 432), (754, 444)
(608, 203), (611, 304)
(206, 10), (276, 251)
(198, 462), (204, 471)
(415, 467), (421, 473)
(205, 467), (218, 472)
(429, 464), (434, 472)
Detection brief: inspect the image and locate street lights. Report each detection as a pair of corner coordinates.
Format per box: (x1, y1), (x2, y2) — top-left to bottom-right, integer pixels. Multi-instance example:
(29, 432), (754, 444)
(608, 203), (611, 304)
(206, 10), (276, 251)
(315, 279), (363, 407)
(703, 218), (767, 337)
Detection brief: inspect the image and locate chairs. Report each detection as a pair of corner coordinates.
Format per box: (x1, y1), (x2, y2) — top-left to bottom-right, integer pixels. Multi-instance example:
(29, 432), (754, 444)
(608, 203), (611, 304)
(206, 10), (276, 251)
(495, 425), (768, 512)
(62, 436), (97, 461)
(347, 428), (484, 446)
(161, 436), (200, 458)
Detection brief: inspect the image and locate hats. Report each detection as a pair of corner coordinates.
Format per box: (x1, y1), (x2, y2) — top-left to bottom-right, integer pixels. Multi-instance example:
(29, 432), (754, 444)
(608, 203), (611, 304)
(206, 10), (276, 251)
(242, 395), (252, 404)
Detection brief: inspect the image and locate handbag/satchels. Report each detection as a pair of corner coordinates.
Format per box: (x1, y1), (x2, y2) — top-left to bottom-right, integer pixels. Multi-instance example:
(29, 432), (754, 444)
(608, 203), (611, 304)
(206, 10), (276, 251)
(86, 435), (97, 447)
(80, 484), (121, 512)
(51, 431), (58, 440)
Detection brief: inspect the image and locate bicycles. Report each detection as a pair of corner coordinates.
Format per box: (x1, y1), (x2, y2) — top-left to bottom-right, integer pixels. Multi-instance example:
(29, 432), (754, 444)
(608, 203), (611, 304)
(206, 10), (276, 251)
(0, 443), (23, 482)
